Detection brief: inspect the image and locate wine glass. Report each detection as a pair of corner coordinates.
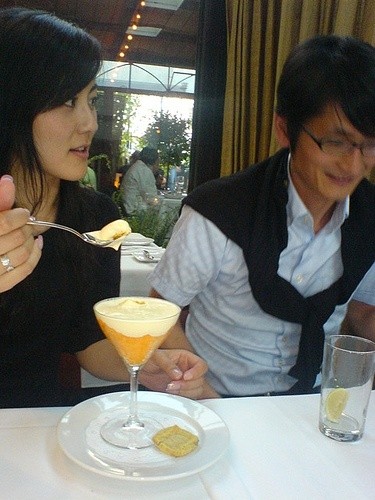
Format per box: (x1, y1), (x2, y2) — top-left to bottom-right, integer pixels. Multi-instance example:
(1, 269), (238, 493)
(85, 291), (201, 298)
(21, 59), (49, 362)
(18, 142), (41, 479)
(94, 296), (181, 449)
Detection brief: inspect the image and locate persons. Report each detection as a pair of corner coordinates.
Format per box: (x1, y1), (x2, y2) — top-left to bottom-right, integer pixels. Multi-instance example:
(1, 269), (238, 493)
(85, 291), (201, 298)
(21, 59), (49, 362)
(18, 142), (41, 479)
(120, 146), (163, 217)
(119, 150), (142, 183)
(149, 33), (375, 402)
(1, 5), (208, 412)
(153, 168), (165, 190)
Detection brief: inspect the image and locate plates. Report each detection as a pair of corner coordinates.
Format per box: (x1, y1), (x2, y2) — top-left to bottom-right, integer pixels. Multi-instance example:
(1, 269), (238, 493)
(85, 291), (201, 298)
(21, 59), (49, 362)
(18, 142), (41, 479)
(120, 237), (165, 263)
(56, 391), (230, 482)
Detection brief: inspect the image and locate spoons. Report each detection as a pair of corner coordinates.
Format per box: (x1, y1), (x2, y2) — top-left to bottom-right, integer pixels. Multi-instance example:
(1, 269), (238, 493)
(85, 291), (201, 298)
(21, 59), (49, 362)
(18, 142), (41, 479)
(26, 219), (130, 247)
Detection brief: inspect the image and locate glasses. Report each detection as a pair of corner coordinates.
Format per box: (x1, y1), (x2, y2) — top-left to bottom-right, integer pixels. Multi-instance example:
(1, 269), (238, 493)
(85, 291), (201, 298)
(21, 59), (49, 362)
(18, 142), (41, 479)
(295, 120), (375, 157)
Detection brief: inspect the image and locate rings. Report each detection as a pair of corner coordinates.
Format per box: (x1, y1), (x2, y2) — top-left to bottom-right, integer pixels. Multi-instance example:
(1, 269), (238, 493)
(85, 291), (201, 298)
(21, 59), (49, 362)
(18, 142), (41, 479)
(0, 252), (15, 275)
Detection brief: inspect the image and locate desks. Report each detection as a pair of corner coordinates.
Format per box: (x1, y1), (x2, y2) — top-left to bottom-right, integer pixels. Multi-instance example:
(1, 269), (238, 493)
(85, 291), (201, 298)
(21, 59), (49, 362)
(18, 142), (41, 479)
(120, 233), (166, 296)
(0, 390), (374, 498)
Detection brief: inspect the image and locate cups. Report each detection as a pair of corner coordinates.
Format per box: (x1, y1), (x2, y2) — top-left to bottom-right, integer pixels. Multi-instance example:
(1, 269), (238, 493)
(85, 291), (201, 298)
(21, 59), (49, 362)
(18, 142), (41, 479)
(319, 335), (375, 442)
